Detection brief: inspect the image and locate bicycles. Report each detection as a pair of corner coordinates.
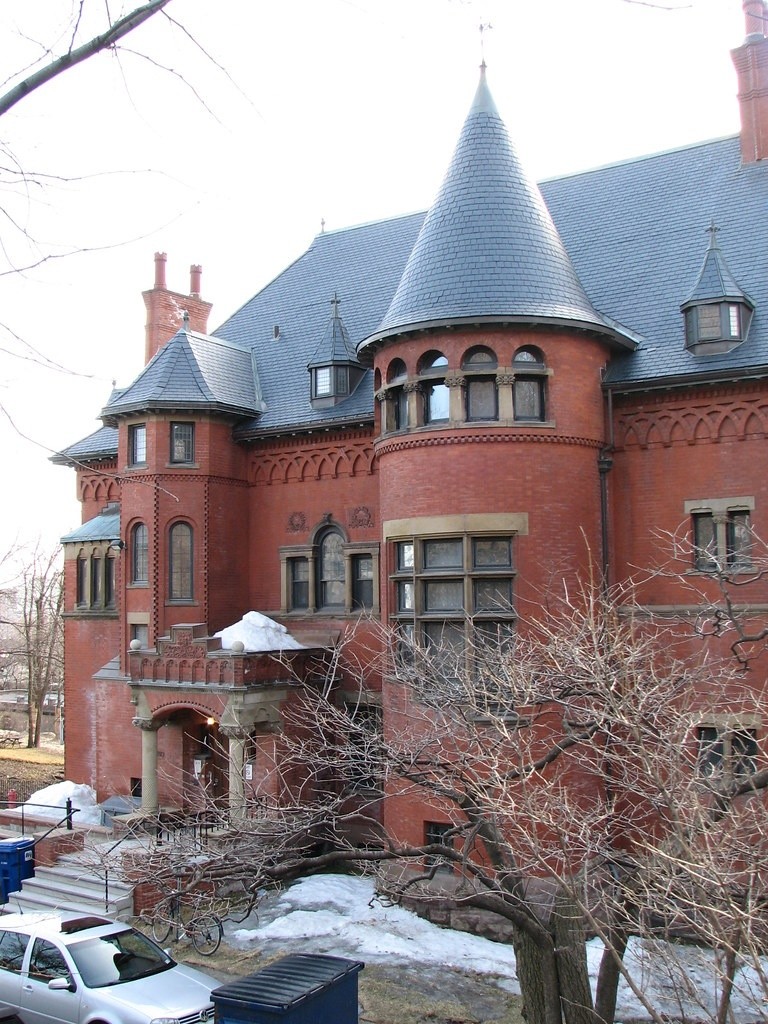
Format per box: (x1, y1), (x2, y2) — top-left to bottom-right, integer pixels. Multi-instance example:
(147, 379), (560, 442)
(152, 887), (224, 957)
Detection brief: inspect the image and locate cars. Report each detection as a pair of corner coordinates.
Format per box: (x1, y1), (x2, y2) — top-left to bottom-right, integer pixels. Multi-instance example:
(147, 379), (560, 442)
(0, 908), (225, 1024)
(42, 693), (64, 715)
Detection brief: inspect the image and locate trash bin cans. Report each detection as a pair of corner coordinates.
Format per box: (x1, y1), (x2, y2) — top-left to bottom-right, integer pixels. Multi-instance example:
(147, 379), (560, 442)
(208, 951), (366, 1024)
(0, 836), (35, 905)
